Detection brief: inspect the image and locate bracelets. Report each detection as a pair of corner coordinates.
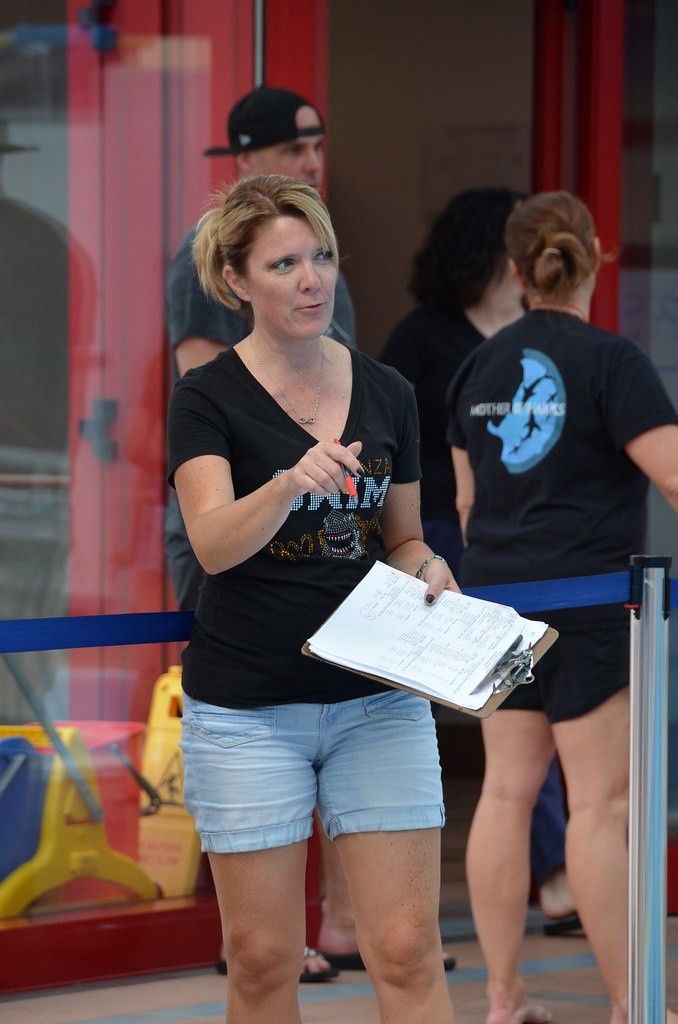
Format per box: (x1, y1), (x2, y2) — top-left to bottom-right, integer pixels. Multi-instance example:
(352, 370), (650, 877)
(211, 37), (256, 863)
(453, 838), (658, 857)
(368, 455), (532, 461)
(416, 554), (448, 581)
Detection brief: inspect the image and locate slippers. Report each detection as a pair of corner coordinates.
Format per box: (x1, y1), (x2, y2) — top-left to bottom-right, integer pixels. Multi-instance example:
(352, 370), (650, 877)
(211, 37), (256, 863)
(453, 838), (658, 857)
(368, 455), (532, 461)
(216, 946), (339, 984)
(319, 946), (458, 973)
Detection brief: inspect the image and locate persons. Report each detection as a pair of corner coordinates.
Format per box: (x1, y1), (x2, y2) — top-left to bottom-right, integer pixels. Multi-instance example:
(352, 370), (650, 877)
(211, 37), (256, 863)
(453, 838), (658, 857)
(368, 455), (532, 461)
(164, 173), (456, 1023)
(375, 188), (588, 933)
(164, 84), (456, 984)
(449, 191), (678, 1024)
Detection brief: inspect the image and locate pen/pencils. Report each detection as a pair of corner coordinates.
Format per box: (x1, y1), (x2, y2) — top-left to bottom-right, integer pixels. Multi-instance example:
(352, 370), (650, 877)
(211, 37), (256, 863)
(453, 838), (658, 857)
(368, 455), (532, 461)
(333, 438), (358, 505)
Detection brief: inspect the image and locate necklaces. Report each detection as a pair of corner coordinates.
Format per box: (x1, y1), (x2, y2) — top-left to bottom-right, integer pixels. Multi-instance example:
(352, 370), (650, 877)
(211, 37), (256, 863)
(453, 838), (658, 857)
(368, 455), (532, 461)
(248, 332), (326, 425)
(529, 299), (591, 326)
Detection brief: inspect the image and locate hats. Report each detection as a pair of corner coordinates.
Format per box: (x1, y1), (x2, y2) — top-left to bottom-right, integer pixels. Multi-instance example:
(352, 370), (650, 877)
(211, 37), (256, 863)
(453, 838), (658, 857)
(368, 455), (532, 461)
(203, 86), (326, 158)
